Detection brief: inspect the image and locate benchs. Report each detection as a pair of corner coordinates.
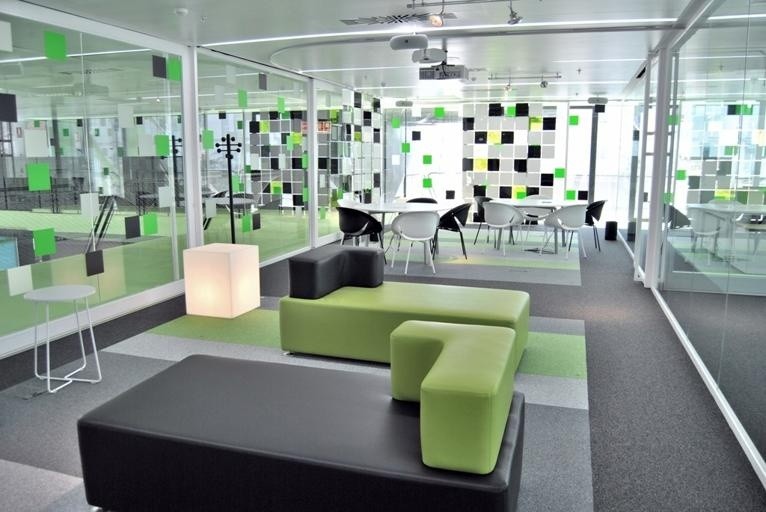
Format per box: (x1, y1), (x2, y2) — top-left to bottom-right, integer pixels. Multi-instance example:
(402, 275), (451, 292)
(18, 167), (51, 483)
(75, 353), (528, 511)
(280, 286), (530, 375)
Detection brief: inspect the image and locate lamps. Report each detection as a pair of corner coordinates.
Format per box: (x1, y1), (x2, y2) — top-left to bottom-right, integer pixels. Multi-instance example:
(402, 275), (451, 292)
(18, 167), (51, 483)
(429, 1), (523, 28)
(485, 68), (562, 93)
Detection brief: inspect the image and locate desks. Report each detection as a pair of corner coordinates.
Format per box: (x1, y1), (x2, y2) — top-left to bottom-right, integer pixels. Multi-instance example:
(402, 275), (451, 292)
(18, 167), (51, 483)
(183, 241), (265, 318)
(22, 286), (105, 392)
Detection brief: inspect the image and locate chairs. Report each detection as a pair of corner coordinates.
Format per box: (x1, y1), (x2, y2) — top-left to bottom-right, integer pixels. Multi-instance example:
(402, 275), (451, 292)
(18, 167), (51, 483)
(198, 171), (290, 235)
(327, 178), (609, 276)
(687, 180), (766, 256)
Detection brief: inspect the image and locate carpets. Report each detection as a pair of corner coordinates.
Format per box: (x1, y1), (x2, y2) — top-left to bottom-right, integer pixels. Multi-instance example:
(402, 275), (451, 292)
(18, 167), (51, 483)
(349, 216), (582, 290)
(2, 292), (597, 511)
(667, 233), (766, 299)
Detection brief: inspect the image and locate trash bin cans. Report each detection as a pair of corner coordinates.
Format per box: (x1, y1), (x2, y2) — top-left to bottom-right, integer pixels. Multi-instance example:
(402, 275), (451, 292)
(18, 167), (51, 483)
(604, 221), (617, 240)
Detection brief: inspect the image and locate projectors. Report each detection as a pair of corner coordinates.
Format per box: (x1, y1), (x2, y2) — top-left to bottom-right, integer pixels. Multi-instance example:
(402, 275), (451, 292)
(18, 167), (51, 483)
(418, 65), (469, 80)
